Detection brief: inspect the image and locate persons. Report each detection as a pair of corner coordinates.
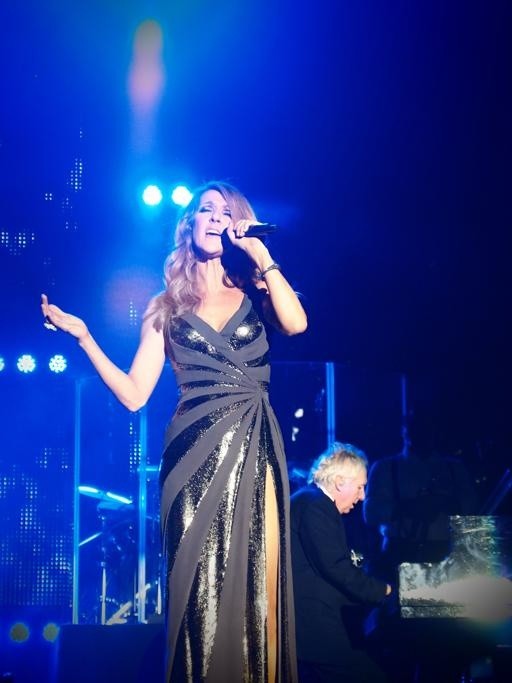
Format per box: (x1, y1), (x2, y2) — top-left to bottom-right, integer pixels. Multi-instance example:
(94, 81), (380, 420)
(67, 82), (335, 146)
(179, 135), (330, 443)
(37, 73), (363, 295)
(358, 399), (480, 682)
(37, 176), (314, 682)
(286, 439), (402, 678)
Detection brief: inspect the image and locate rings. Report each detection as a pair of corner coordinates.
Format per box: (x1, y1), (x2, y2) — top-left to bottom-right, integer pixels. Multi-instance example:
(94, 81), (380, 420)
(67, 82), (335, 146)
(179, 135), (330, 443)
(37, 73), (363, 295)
(44, 317), (60, 334)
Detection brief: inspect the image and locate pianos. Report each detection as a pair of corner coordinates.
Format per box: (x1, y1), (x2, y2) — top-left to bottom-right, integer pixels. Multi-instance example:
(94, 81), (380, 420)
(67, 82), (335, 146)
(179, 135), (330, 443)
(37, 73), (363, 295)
(399, 461), (511, 682)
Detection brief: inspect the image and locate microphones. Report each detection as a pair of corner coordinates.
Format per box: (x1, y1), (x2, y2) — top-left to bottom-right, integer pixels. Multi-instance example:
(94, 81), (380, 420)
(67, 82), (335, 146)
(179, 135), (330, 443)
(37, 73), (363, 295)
(234, 222), (278, 237)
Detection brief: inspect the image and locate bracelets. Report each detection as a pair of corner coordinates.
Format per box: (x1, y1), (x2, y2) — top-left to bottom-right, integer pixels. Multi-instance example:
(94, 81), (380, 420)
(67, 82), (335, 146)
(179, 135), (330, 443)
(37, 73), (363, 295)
(260, 261), (282, 279)
